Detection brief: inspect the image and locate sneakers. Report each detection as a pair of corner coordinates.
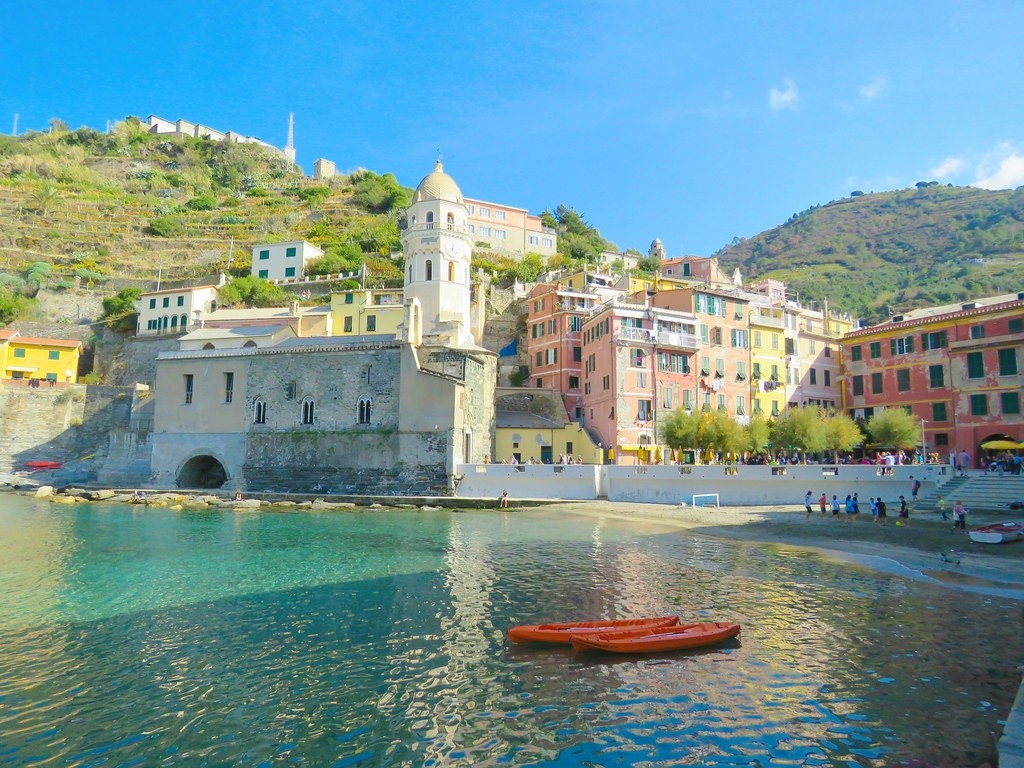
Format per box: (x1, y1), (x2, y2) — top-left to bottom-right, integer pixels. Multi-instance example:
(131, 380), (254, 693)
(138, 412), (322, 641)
(960, 472), (964, 476)
(964, 475), (967, 476)
(960, 530), (967, 534)
(950, 527), (956, 534)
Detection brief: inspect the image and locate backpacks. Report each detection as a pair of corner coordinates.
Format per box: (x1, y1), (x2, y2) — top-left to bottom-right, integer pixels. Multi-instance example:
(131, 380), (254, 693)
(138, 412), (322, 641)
(916, 479), (920, 488)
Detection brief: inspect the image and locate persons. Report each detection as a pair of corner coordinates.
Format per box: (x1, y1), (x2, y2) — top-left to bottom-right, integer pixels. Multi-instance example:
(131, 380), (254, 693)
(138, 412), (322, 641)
(898, 495), (910, 527)
(817, 493), (827, 520)
(231, 488), (242, 502)
(500, 489), (509, 508)
(130, 489), (147, 504)
(829, 495), (840, 521)
(874, 497), (887, 526)
(735, 448), (1024, 479)
(935, 496), (970, 535)
(867, 498), (878, 524)
(851, 492), (861, 522)
(844, 494), (858, 523)
(909, 475), (920, 503)
(483, 452), (582, 473)
(804, 490), (813, 521)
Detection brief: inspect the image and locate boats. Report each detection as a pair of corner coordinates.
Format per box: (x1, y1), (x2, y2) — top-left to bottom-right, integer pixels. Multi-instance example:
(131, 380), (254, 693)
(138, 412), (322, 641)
(25, 460), (62, 467)
(969, 521), (1024, 544)
(27, 466), (61, 471)
(507, 616), (685, 649)
(569, 621), (741, 656)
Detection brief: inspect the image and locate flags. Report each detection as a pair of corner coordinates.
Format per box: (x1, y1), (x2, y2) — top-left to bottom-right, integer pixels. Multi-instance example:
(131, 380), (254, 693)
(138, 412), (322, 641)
(499, 338), (518, 358)
